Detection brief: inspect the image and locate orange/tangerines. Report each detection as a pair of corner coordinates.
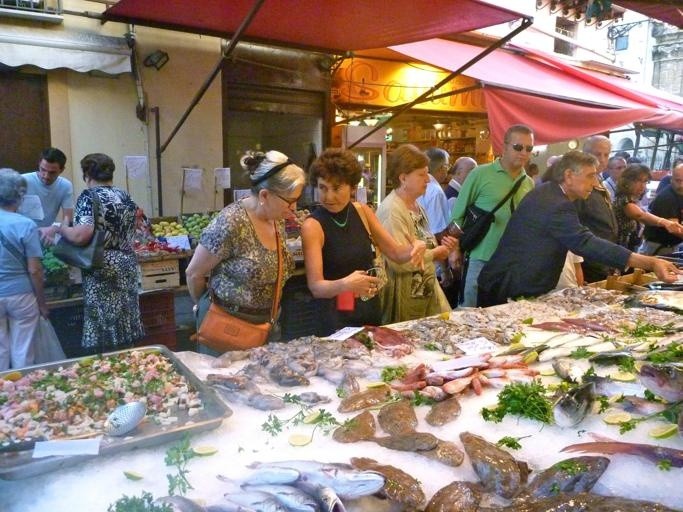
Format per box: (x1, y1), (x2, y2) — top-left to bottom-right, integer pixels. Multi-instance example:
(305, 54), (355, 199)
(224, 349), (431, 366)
(181, 214), (210, 239)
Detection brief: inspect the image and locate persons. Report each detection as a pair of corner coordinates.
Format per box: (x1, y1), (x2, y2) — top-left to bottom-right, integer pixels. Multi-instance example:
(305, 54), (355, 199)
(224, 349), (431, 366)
(18, 147), (72, 236)
(0, 167), (50, 371)
(299, 147), (428, 339)
(183, 151), (305, 359)
(34, 153), (146, 354)
(371, 145), (477, 326)
(454, 125), (682, 309)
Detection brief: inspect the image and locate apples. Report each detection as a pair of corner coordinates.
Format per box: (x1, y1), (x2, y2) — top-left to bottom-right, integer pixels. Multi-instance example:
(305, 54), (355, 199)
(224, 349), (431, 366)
(151, 220), (190, 238)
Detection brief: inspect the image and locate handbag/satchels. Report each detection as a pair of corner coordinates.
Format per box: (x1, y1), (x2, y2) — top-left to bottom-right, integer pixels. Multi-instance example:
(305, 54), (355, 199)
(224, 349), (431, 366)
(190, 302), (273, 357)
(462, 203), (495, 252)
(433, 221), (463, 247)
(373, 256), (387, 291)
(52, 227), (107, 271)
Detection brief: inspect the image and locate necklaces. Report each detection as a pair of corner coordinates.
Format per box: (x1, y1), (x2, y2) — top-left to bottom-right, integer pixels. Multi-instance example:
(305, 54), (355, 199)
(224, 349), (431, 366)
(325, 205), (351, 227)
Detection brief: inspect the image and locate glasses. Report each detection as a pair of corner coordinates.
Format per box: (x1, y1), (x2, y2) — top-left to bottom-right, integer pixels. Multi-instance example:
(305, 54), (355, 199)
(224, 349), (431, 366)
(511, 143), (534, 152)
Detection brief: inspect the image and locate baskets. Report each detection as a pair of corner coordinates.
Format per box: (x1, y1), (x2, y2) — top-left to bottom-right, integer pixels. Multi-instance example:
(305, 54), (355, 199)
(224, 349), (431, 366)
(279, 274), (336, 340)
(133, 291), (179, 351)
(48, 305), (100, 358)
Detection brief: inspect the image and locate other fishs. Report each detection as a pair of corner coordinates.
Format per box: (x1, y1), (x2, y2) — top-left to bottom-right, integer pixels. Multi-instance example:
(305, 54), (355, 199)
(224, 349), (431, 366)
(386, 353), (540, 401)
(331, 401), (677, 510)
(363, 322), (416, 352)
(202, 325), (367, 411)
(215, 457), (389, 512)
(561, 433), (683, 474)
(519, 320), (683, 427)
